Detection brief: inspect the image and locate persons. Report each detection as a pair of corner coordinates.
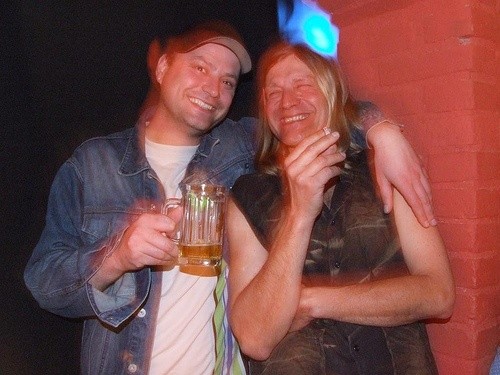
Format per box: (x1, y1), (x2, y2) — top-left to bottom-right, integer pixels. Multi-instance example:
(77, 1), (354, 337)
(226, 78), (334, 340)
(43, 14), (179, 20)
(24, 19), (438, 375)
(226, 40), (456, 375)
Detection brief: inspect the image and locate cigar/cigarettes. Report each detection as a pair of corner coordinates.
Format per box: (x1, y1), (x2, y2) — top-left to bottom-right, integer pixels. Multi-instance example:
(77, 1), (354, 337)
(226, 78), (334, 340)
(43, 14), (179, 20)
(323, 127), (332, 136)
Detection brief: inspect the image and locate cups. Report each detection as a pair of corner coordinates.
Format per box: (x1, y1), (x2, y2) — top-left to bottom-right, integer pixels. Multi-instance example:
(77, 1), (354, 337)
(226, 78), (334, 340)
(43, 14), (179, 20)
(159, 183), (228, 277)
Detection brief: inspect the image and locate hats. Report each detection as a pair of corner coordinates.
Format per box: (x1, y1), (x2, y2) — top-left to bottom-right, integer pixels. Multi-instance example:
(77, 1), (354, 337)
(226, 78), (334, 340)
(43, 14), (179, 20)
(168, 16), (252, 74)
